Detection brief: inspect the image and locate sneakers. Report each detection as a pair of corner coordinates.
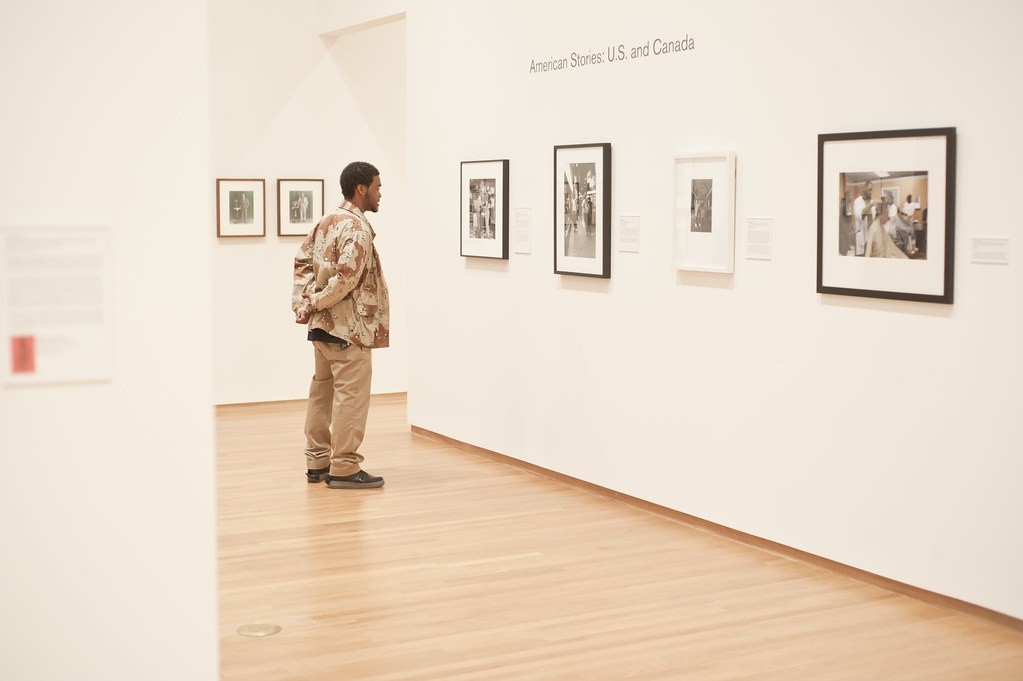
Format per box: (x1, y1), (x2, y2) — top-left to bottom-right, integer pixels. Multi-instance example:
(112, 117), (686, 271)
(305, 465), (330, 482)
(325, 469), (384, 489)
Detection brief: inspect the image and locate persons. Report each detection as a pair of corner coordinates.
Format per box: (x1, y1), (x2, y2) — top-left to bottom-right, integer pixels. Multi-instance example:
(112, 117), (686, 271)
(240, 193), (249, 223)
(694, 199), (706, 228)
(852, 180), (920, 259)
(470, 180), (495, 239)
(291, 161), (389, 488)
(569, 193), (593, 236)
(297, 192), (308, 222)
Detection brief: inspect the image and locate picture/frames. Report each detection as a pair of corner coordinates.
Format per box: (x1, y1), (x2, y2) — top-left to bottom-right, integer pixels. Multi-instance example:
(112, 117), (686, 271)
(276, 177), (326, 239)
(669, 150), (739, 276)
(810, 125), (959, 305)
(215, 175), (266, 238)
(551, 140), (615, 282)
(455, 156), (511, 262)
